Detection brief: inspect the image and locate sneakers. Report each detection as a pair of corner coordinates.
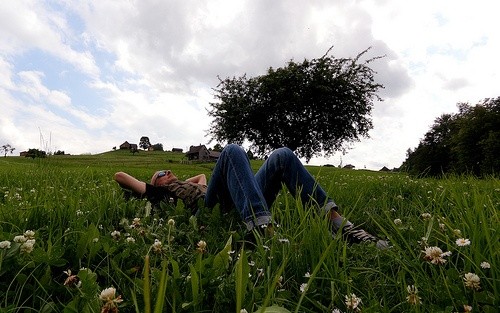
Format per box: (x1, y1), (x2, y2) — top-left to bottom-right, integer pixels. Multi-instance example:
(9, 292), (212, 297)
(332, 225), (389, 248)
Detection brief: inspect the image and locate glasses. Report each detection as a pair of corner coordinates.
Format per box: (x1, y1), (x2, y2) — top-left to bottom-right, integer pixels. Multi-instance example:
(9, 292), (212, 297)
(155, 170), (168, 185)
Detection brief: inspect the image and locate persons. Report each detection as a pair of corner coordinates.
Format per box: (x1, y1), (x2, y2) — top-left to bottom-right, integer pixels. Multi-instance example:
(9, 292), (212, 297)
(111, 142), (390, 258)
